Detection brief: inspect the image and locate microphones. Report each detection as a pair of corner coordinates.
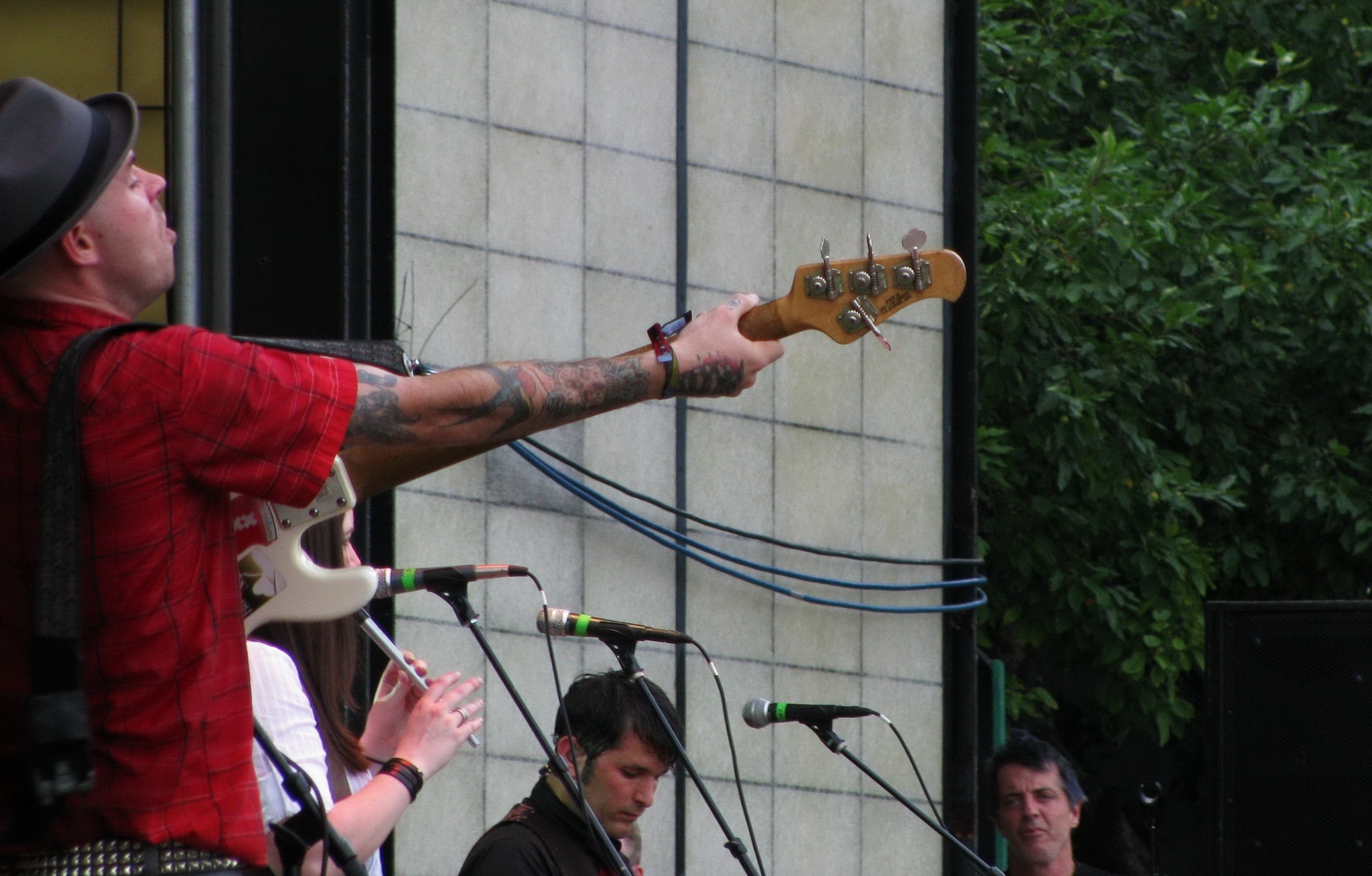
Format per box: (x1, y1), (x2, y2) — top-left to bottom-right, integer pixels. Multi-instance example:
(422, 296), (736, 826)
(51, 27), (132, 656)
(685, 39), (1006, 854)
(742, 697), (872, 729)
(536, 608), (692, 645)
(374, 565), (530, 598)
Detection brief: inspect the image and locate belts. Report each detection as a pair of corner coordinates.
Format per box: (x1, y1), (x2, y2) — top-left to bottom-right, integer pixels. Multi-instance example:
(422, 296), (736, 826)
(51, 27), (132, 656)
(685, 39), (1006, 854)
(0, 832), (244, 876)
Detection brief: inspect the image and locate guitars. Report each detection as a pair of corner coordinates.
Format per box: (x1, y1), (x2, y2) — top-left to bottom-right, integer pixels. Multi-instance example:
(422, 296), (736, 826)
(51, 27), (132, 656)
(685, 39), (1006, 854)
(235, 225), (968, 639)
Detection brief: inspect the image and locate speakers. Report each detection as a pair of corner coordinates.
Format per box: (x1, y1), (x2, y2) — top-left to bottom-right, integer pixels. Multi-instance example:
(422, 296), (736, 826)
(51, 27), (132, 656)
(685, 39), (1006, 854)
(1204, 598), (1372, 876)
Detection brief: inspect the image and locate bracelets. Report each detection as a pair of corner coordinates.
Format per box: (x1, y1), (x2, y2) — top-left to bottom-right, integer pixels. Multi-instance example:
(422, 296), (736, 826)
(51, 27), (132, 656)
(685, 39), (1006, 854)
(647, 310), (692, 400)
(377, 758), (424, 804)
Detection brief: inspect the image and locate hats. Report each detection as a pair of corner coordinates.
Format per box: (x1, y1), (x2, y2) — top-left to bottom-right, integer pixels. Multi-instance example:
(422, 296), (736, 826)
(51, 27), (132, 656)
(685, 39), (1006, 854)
(0, 73), (139, 281)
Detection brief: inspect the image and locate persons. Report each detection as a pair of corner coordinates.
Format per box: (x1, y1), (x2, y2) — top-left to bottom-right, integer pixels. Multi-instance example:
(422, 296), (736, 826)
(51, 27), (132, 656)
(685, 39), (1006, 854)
(456, 668), (685, 876)
(988, 735), (1118, 876)
(239, 505), (487, 876)
(0, 77), (787, 876)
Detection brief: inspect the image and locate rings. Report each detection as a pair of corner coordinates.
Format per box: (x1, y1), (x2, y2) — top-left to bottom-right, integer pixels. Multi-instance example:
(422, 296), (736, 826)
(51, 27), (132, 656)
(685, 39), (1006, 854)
(455, 708), (470, 722)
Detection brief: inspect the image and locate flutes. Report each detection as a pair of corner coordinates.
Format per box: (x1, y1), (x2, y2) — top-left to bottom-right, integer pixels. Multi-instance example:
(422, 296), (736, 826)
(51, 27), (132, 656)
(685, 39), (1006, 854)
(351, 607), (482, 749)
